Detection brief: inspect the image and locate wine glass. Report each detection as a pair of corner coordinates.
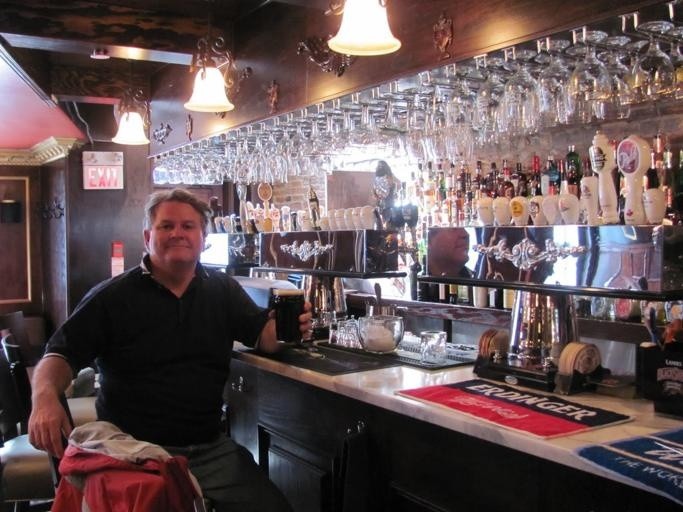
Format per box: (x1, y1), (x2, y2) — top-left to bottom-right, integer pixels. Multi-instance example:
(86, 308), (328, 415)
(151, 20), (683, 186)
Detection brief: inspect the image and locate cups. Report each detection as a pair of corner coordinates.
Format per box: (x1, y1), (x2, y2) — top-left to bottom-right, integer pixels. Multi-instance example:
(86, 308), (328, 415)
(419, 330), (447, 365)
(337, 320), (359, 350)
(273, 287), (305, 344)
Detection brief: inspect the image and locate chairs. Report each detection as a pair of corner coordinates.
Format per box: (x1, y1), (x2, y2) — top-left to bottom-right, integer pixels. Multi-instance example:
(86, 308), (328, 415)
(0, 309), (100, 512)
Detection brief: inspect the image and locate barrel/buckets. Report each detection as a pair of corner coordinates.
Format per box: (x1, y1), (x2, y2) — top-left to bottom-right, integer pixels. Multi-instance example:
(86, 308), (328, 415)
(300, 275), (349, 316)
(507, 291), (579, 373)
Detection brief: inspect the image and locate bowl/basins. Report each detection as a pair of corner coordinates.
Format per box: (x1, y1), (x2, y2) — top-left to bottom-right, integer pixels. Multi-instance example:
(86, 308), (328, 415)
(358, 315), (404, 354)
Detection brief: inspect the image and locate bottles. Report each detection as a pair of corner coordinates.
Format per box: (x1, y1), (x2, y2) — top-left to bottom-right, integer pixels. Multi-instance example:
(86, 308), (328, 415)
(329, 324), (338, 344)
(210, 126), (683, 233)
(655, 318), (683, 415)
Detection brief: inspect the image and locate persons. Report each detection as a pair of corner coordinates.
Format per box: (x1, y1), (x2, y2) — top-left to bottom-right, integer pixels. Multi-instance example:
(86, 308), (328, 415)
(28, 190), (314, 511)
(417, 228), (477, 303)
